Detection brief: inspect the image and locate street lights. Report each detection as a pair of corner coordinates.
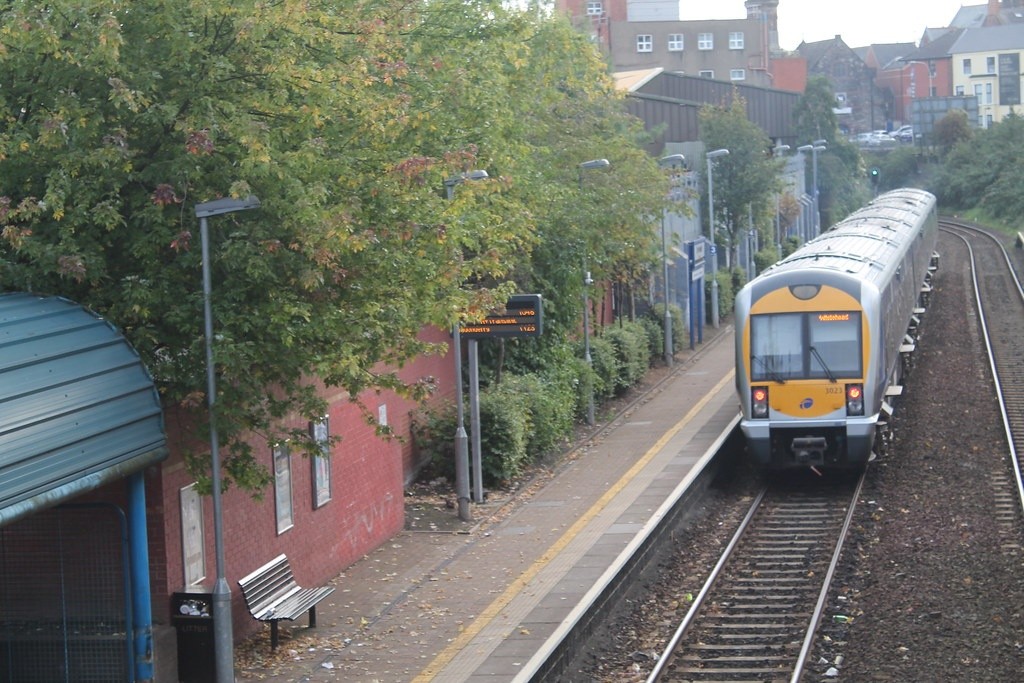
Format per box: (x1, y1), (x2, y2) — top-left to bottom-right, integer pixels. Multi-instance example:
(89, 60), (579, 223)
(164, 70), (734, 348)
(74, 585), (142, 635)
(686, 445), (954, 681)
(705, 148), (729, 327)
(773, 145), (790, 259)
(442, 171), (488, 521)
(195, 195), (261, 683)
(576, 158), (610, 425)
(658, 154), (687, 366)
(797, 140), (827, 247)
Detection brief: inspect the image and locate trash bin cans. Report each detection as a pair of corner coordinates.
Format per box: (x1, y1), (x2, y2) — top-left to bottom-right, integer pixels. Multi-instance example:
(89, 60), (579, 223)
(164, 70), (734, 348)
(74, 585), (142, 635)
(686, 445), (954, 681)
(170, 591), (218, 683)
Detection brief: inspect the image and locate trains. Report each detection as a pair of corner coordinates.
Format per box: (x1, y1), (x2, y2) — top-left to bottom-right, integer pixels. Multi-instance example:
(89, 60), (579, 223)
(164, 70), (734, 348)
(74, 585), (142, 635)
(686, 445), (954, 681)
(735, 187), (940, 494)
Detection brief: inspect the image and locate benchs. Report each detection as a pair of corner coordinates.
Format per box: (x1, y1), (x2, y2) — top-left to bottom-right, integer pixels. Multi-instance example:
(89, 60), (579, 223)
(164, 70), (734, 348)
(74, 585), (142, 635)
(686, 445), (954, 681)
(238, 553), (336, 652)
